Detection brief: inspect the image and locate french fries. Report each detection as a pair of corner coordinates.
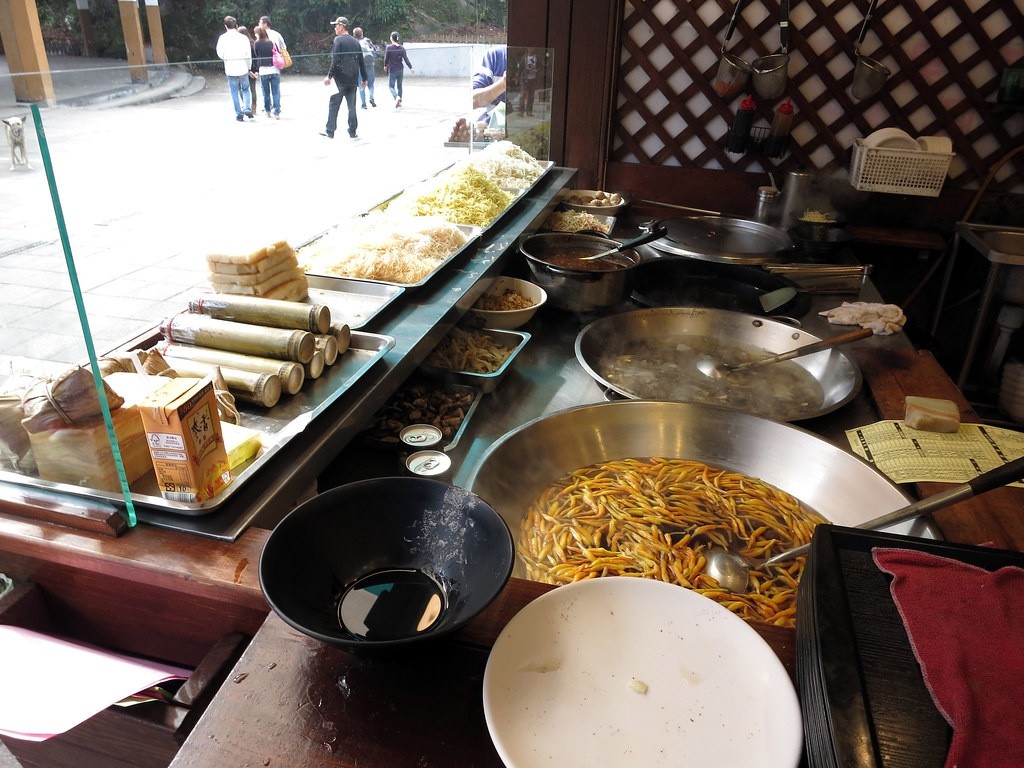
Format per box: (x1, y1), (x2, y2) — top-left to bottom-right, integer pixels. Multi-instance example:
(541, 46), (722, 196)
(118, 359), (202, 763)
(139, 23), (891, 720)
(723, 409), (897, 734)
(431, 329), (518, 374)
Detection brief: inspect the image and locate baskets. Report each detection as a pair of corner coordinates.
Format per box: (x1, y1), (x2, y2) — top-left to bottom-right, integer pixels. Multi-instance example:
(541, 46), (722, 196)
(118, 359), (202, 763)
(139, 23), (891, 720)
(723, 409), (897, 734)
(848, 135), (956, 197)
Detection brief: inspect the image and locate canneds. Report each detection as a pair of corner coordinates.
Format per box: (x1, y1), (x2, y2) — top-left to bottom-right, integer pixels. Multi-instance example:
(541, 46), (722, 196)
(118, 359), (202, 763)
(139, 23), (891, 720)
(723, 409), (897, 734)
(405, 450), (453, 485)
(397, 424), (444, 477)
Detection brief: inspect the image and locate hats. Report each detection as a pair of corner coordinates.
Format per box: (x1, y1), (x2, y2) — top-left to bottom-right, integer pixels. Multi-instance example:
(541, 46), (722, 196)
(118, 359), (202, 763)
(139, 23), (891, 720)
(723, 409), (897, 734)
(330, 17), (349, 26)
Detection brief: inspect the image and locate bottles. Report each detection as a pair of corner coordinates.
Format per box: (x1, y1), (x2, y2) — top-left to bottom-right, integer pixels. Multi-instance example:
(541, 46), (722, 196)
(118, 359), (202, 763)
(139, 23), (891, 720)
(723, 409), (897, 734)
(780, 171), (814, 226)
(754, 185), (779, 224)
(765, 97), (794, 157)
(728, 95), (755, 154)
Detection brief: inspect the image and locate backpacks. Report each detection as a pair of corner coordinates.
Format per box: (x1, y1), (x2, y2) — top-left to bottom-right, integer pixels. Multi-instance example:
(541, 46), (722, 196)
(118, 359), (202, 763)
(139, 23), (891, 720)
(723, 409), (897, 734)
(359, 37), (377, 64)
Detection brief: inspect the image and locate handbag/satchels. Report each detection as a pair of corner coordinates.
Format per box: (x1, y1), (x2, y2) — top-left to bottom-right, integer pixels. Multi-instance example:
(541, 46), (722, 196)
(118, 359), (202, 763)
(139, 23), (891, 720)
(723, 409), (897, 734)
(272, 40), (285, 69)
(281, 49), (292, 67)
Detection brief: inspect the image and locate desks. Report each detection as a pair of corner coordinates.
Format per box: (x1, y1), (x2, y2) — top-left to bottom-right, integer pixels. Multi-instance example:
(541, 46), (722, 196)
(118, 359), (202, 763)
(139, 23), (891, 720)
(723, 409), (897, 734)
(166, 276), (1024, 768)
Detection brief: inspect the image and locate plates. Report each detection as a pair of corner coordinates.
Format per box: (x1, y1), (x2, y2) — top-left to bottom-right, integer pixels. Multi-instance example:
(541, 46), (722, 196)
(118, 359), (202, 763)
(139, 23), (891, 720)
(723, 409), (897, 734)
(483, 576), (804, 768)
(862, 129), (952, 181)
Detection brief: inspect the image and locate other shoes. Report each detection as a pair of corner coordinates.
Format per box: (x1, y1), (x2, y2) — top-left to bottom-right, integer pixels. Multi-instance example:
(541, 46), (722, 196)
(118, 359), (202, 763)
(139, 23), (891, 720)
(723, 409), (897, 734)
(266, 112), (271, 118)
(245, 112), (253, 118)
(370, 99), (376, 107)
(251, 105), (256, 113)
(274, 116), (279, 120)
(361, 106), (366, 111)
(350, 134), (358, 138)
(319, 132), (333, 138)
(517, 113), (523, 117)
(236, 116), (243, 121)
(395, 96), (402, 108)
(527, 115), (534, 117)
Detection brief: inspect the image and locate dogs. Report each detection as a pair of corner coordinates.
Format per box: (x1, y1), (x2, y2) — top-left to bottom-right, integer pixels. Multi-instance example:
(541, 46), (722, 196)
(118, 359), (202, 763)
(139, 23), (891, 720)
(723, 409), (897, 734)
(2, 117), (34, 171)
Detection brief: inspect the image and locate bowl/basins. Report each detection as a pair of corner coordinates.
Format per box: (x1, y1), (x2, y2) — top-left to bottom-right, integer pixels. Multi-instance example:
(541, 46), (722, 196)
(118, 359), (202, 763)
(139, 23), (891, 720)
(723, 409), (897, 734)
(260, 475), (514, 653)
(787, 224), (853, 262)
(789, 208), (850, 225)
(467, 275), (548, 329)
(561, 189), (627, 216)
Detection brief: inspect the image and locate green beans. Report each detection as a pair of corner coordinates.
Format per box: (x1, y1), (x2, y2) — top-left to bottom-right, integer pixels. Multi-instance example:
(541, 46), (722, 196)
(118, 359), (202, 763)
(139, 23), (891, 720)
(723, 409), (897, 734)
(518, 457), (833, 629)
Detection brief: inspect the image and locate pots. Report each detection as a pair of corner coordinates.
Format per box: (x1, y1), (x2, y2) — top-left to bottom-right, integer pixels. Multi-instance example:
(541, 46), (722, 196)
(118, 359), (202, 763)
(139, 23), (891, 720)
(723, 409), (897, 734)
(465, 399), (944, 628)
(624, 257), (811, 318)
(574, 305), (862, 422)
(519, 229), (643, 313)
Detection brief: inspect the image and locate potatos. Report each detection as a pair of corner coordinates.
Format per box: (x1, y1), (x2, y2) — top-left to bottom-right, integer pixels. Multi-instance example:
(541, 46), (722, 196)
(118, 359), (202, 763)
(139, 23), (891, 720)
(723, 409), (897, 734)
(568, 190), (622, 206)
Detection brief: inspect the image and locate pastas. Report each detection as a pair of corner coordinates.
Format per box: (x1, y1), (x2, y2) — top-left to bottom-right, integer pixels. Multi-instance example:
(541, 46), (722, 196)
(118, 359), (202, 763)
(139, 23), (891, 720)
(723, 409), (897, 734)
(468, 140), (546, 189)
(403, 164), (515, 228)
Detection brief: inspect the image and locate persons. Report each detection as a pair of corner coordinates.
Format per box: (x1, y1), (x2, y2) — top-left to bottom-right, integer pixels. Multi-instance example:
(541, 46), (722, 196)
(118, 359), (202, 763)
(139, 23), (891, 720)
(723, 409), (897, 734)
(319, 17), (367, 140)
(353, 28), (377, 109)
(259, 16), (286, 112)
(237, 26), (257, 114)
(254, 26), (281, 119)
(473, 48), (507, 123)
(384, 32), (414, 108)
(216, 16), (254, 121)
(514, 48), (542, 118)
